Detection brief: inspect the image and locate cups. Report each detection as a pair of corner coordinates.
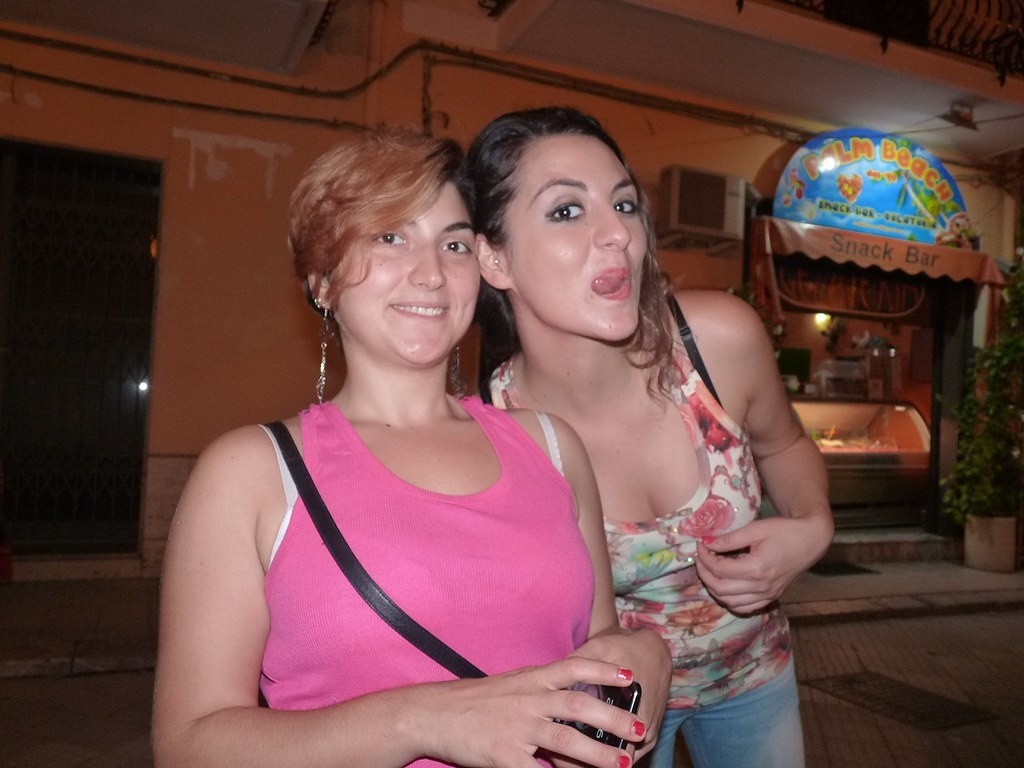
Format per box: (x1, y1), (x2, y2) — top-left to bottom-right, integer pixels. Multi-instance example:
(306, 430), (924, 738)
(805, 383), (815, 395)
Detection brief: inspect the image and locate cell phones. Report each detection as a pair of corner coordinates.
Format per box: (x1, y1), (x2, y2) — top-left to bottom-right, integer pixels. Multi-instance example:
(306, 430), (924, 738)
(552, 680), (642, 750)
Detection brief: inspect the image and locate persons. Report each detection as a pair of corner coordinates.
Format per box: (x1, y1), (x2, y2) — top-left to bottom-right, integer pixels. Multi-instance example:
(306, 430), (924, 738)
(152, 122), (675, 768)
(465, 105), (836, 768)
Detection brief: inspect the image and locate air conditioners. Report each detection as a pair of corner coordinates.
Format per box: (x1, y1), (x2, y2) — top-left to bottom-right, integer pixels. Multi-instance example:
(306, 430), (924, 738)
(656, 163), (746, 244)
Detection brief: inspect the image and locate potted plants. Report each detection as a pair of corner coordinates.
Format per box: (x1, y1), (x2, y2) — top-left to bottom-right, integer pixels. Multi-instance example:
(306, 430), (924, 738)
(938, 245), (1024, 572)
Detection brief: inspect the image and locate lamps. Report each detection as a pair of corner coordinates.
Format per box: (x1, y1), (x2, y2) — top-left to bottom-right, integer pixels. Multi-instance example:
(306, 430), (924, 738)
(937, 94), (979, 130)
